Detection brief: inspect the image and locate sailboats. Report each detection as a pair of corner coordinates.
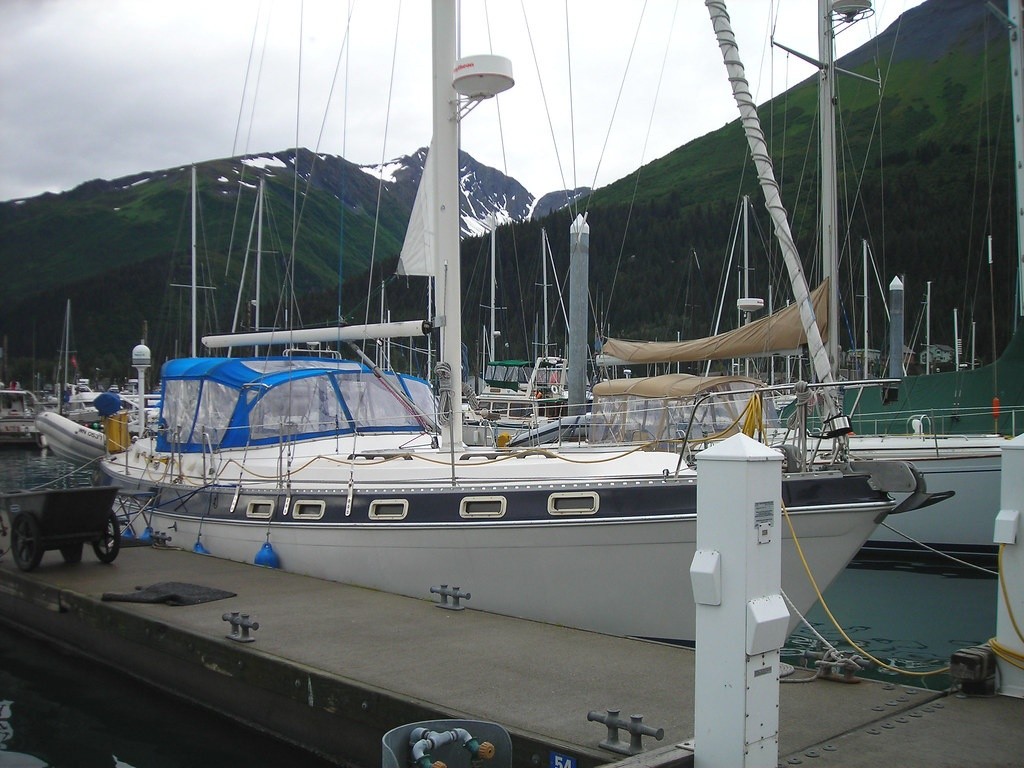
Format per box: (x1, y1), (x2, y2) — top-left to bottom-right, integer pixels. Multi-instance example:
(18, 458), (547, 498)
(1, 3), (1004, 652)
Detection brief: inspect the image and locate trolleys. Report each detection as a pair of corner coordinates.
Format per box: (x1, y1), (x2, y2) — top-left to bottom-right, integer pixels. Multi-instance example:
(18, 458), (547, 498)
(1, 486), (122, 573)
